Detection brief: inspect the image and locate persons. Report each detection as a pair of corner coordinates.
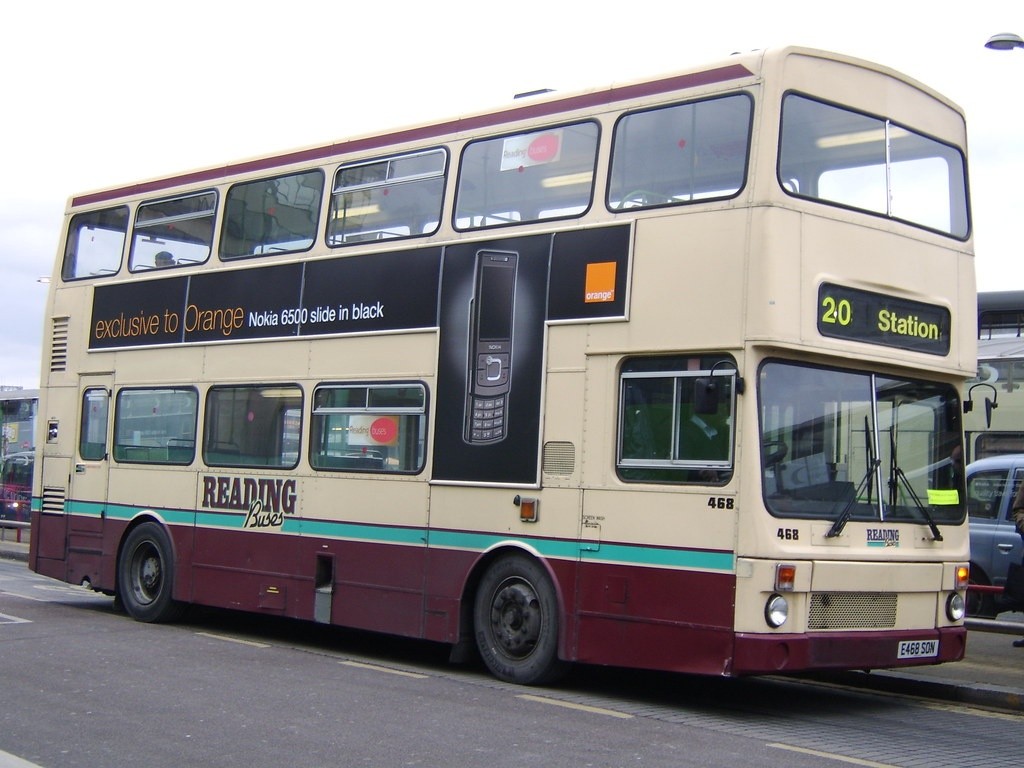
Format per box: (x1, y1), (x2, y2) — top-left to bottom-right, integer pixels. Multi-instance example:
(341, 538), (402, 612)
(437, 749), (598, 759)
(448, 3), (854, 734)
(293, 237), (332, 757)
(689, 376), (733, 487)
(155, 251), (176, 267)
(1011, 480), (1024, 647)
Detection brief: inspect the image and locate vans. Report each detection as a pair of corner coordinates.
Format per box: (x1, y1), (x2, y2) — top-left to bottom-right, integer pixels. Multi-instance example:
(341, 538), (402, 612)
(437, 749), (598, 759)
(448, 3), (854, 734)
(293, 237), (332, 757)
(964, 453), (1024, 622)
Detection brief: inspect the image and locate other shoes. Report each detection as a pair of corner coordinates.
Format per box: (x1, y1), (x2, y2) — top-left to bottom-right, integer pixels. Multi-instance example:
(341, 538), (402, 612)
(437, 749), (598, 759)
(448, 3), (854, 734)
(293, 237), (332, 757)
(1012, 638), (1024, 647)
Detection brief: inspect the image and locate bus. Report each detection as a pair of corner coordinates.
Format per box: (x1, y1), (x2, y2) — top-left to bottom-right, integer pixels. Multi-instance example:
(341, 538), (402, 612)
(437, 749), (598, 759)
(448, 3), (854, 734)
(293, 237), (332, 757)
(27, 51), (1000, 682)
(0, 451), (33, 522)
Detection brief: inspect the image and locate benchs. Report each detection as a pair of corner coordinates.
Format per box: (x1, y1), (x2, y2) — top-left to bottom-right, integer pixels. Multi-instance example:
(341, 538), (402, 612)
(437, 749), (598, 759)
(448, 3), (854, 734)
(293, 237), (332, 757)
(99, 444), (143, 459)
(166, 438), (241, 464)
(123, 447), (167, 461)
(317, 449), (386, 469)
